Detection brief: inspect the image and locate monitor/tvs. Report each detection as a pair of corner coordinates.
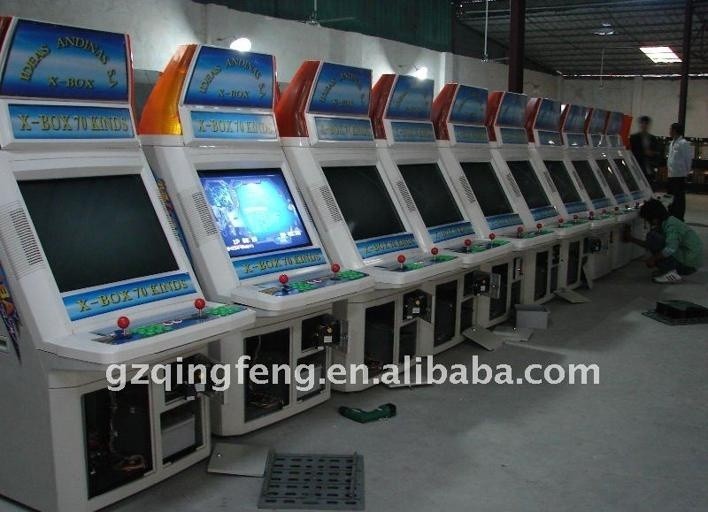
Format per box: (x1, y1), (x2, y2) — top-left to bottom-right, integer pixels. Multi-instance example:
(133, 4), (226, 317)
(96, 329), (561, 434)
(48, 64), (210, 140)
(570, 160), (606, 200)
(397, 162), (464, 228)
(543, 160), (582, 203)
(321, 165), (407, 241)
(613, 159), (640, 192)
(459, 161), (514, 217)
(17, 173), (180, 294)
(197, 168), (312, 258)
(506, 161), (551, 210)
(595, 159), (625, 197)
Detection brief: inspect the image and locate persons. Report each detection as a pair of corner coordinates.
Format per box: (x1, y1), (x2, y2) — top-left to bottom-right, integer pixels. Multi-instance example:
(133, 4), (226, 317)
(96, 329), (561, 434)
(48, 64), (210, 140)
(628, 116), (661, 194)
(624, 198), (704, 283)
(666, 123), (694, 223)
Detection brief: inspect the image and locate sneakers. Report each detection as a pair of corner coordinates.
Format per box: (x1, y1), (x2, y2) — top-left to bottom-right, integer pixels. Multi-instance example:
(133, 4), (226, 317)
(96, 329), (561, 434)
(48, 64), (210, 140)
(652, 270), (682, 282)
